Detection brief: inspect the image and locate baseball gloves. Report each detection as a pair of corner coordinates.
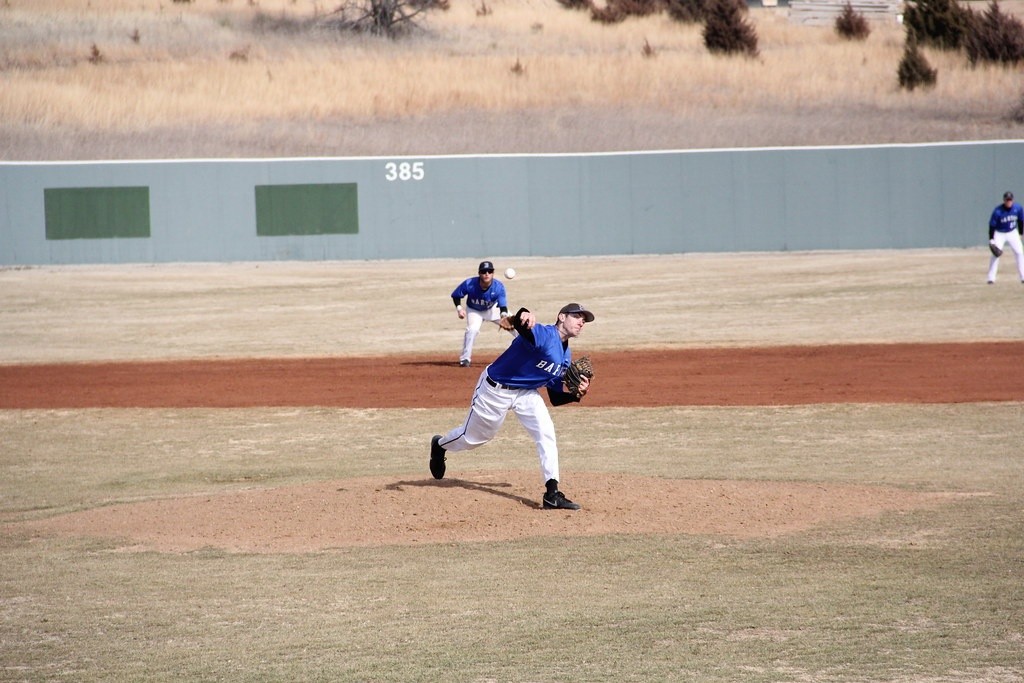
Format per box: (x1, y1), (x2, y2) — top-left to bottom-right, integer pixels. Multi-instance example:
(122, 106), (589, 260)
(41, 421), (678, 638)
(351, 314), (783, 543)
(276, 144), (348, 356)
(561, 355), (593, 399)
(990, 243), (1003, 257)
(498, 314), (516, 331)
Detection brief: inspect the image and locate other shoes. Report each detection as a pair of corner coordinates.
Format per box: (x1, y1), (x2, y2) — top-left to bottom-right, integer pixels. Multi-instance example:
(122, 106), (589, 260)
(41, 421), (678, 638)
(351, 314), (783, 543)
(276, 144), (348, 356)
(460, 359), (470, 367)
(988, 281), (994, 284)
(1021, 281), (1024, 283)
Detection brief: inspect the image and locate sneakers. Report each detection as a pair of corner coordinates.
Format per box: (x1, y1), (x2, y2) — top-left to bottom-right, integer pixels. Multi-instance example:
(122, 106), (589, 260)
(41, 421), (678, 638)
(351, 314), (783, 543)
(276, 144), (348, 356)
(543, 490), (580, 510)
(429, 435), (448, 480)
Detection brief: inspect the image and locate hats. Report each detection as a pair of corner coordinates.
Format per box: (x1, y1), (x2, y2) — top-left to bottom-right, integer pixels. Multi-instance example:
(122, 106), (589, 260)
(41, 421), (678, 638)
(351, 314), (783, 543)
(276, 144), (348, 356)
(1003, 192), (1014, 200)
(558, 303), (594, 322)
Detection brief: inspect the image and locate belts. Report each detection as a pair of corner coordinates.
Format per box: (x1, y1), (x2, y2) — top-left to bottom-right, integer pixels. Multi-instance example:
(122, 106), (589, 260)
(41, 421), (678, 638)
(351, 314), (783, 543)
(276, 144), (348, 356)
(486, 376), (521, 390)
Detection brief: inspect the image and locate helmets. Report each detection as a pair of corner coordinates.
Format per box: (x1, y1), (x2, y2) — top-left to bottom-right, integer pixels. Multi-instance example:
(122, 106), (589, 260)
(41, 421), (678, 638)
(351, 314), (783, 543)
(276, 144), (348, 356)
(479, 261), (494, 270)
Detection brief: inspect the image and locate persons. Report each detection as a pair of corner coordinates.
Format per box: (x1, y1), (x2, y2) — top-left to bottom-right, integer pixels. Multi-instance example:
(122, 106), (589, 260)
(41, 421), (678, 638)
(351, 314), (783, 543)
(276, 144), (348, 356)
(451, 261), (519, 367)
(430, 303), (594, 509)
(987, 192), (1024, 285)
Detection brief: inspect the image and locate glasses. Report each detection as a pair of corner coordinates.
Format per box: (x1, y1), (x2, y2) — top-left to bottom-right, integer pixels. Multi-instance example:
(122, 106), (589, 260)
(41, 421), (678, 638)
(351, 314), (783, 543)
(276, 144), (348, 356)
(479, 269), (494, 274)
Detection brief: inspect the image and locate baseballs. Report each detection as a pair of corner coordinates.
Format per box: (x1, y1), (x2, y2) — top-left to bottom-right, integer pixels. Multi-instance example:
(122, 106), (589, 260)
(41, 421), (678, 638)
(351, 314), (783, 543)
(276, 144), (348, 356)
(503, 268), (516, 280)
